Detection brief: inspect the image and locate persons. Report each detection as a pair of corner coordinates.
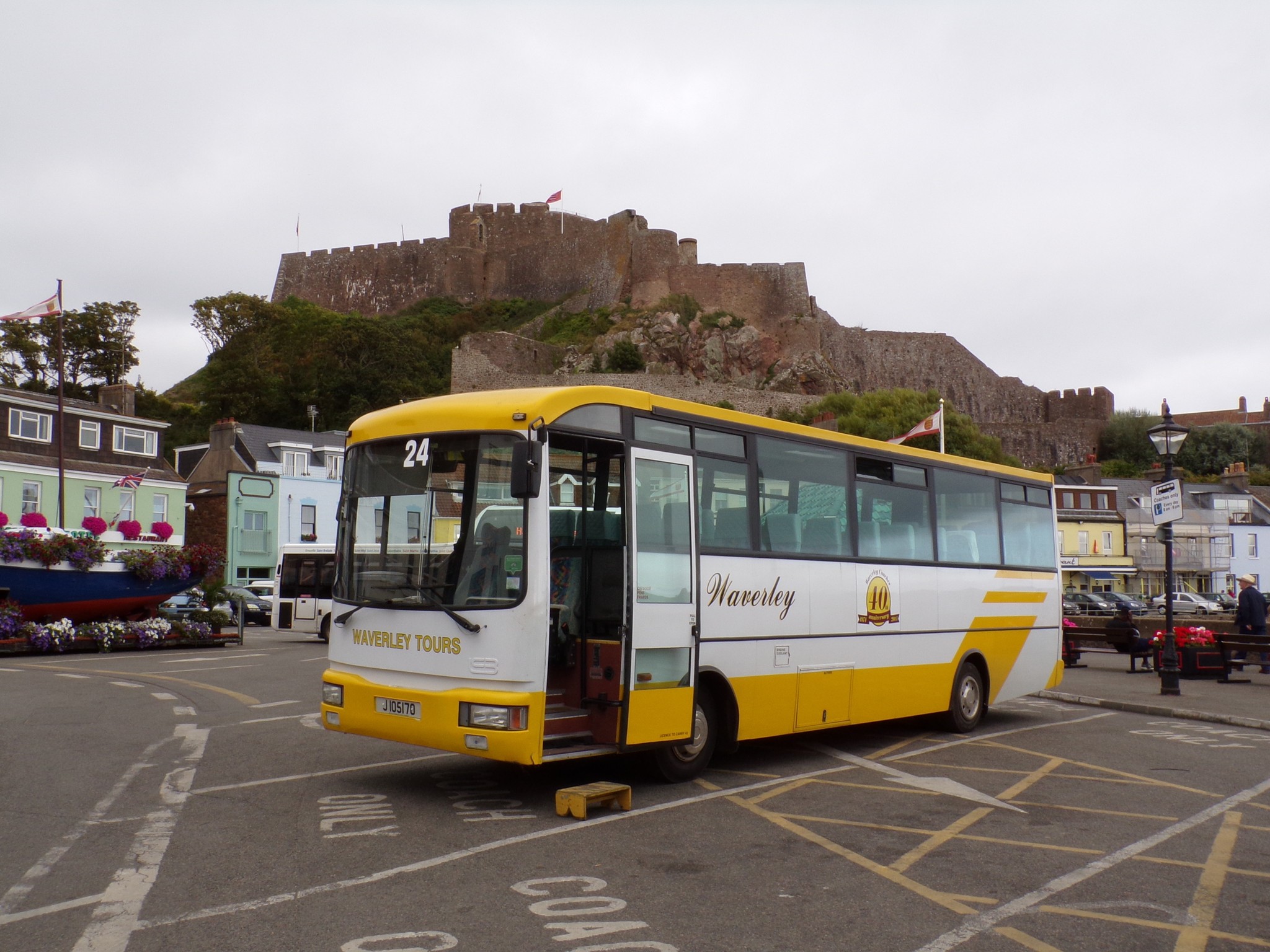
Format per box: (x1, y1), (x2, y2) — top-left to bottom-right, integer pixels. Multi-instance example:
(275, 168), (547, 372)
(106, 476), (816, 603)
(1221, 589), (1236, 598)
(1106, 606), (1154, 671)
(1235, 574), (1270, 674)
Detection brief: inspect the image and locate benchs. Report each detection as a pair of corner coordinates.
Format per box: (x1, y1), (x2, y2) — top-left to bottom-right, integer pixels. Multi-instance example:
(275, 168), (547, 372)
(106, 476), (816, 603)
(1212, 634), (1270, 683)
(1062, 625), (1154, 673)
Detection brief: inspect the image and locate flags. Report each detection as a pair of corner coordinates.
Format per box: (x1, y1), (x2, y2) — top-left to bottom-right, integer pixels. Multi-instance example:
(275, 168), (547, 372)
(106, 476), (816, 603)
(886, 409), (942, 445)
(108, 471), (147, 490)
(297, 216), (299, 236)
(0, 288), (62, 321)
(478, 188), (481, 201)
(545, 190), (562, 203)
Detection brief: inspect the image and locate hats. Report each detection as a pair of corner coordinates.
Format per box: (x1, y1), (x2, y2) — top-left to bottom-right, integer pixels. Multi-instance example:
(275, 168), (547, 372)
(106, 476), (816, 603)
(1235, 574), (1257, 586)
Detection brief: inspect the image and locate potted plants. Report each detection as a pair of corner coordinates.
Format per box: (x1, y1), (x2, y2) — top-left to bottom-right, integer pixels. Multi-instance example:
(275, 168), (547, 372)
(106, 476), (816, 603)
(185, 578), (252, 634)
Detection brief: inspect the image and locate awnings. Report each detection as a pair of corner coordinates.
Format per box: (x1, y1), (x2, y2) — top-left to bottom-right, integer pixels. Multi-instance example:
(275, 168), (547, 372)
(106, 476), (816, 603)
(1061, 565), (1138, 581)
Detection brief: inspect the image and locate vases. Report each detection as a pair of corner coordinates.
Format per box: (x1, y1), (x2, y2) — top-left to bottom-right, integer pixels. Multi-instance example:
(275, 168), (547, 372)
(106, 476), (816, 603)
(1151, 644), (1230, 680)
(1060, 638), (1081, 664)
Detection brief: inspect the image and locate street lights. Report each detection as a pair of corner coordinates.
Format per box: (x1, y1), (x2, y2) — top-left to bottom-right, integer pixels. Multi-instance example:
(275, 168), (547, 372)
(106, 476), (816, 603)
(1146, 407), (1191, 696)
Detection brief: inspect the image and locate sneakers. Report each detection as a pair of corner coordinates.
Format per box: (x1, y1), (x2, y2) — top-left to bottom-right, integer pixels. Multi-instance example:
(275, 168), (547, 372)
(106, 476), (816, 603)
(1140, 661), (1154, 670)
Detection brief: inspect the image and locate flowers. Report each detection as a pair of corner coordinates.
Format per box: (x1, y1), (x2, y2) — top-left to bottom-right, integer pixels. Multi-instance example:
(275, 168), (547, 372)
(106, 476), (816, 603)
(1063, 618), (1079, 627)
(1148, 626), (1227, 648)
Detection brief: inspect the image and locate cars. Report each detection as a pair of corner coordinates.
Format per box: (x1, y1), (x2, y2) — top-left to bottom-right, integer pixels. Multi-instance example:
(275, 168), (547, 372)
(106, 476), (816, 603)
(1153, 592), (1224, 615)
(159, 581), (274, 629)
(1064, 591), (1270, 618)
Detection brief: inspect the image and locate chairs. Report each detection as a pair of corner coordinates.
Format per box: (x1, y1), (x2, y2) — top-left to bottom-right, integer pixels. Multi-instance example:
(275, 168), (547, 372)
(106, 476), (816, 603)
(549, 503), (979, 631)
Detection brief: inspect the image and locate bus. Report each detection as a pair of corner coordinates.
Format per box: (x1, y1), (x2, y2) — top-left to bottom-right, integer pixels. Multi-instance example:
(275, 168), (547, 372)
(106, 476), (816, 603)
(270, 543), (521, 644)
(473, 504), (621, 547)
(317, 386), (1066, 785)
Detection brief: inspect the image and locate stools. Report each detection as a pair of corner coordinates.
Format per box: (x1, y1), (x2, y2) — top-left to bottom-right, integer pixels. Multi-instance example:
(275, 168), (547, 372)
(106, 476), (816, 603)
(555, 781), (631, 821)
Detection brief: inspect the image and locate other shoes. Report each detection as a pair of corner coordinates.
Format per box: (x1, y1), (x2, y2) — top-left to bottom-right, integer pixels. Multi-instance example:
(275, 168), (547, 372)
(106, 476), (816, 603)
(1230, 665), (1244, 671)
(1258, 668), (1270, 674)
